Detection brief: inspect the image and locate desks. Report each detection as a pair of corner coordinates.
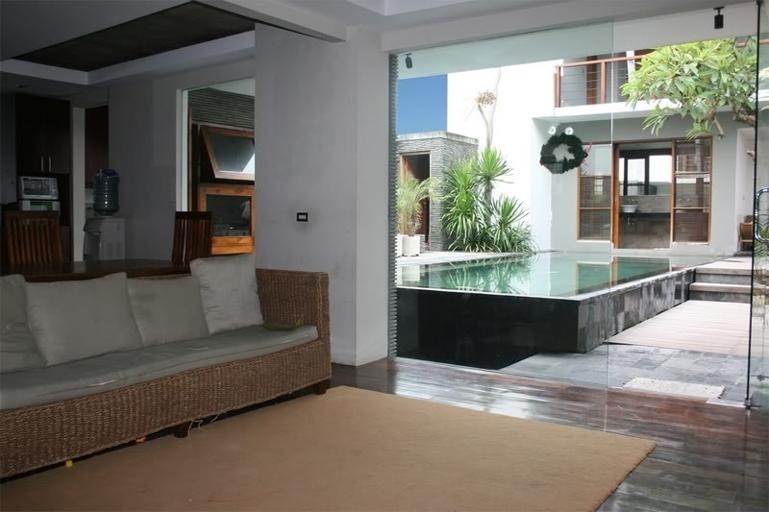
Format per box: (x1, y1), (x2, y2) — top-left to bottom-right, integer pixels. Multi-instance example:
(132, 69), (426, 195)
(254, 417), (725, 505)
(0, 257), (192, 284)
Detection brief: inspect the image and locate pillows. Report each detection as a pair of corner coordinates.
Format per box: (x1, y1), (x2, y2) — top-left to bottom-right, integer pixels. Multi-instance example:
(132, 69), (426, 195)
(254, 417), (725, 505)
(124, 273), (209, 349)
(23, 271), (145, 367)
(0, 273), (47, 374)
(187, 252), (265, 338)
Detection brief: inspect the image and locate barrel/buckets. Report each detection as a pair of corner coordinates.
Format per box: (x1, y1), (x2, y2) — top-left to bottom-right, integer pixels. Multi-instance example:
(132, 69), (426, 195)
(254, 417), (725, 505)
(94, 168), (120, 216)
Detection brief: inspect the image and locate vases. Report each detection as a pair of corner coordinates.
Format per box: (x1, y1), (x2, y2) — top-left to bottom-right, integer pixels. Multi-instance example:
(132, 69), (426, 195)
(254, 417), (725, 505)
(404, 265), (420, 287)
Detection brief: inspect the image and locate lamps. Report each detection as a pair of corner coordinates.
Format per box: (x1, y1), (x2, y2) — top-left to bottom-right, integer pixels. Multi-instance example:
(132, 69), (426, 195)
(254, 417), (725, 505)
(404, 53), (415, 67)
(711, 3), (725, 29)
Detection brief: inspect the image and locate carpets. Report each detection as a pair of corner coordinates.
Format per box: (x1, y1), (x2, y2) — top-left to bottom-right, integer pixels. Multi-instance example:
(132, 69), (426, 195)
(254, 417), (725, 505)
(620, 377), (725, 403)
(2, 383), (658, 512)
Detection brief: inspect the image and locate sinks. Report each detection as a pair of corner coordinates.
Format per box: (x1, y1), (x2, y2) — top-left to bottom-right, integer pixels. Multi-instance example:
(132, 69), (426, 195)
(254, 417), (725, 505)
(620, 204), (637, 213)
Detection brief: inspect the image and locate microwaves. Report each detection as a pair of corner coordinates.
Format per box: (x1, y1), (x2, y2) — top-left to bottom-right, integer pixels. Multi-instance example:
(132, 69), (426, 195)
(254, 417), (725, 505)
(18, 200), (61, 215)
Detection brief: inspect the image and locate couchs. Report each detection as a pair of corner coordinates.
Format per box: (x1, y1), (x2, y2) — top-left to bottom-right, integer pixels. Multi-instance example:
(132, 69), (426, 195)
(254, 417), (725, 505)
(1, 266), (336, 481)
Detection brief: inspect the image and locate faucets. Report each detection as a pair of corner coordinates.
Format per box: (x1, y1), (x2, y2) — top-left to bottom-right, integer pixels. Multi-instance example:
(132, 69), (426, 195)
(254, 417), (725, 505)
(631, 199), (635, 204)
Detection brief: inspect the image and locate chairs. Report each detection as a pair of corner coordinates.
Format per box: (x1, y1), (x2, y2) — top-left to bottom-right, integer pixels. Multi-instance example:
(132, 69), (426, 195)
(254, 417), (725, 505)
(168, 210), (214, 266)
(3, 209), (65, 268)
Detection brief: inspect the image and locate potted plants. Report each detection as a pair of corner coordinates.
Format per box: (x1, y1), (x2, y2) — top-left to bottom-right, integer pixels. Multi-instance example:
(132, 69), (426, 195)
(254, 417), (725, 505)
(396, 182), (403, 258)
(397, 175), (446, 257)
